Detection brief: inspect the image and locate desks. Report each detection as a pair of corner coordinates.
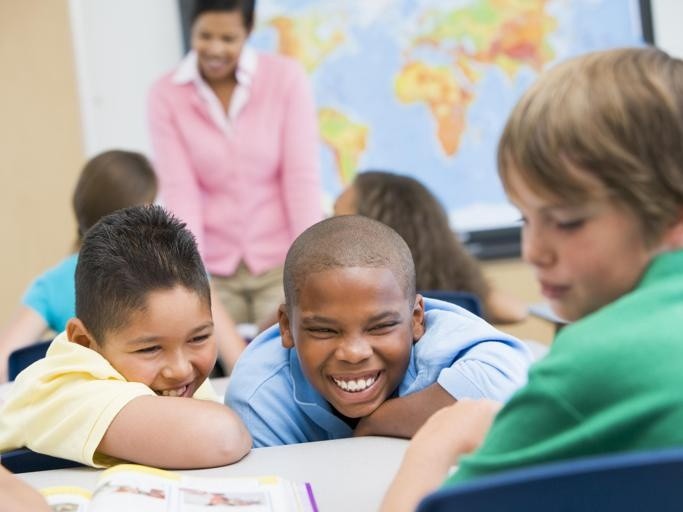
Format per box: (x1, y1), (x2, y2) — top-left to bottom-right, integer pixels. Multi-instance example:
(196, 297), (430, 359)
(0, 428), (462, 510)
(525, 303), (568, 341)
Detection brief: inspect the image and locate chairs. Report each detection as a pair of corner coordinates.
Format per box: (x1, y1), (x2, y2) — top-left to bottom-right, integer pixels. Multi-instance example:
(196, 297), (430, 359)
(417, 288), (485, 318)
(414, 446), (682, 511)
(0, 444), (88, 475)
(7, 341), (55, 381)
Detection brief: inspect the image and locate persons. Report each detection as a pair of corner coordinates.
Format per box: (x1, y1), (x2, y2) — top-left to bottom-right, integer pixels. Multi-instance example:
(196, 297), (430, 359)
(0, 204), (253, 470)
(376, 48), (683, 512)
(0, 463), (54, 512)
(0, 149), (246, 385)
(256, 170), (529, 333)
(223, 213), (535, 449)
(141, 2), (329, 326)
